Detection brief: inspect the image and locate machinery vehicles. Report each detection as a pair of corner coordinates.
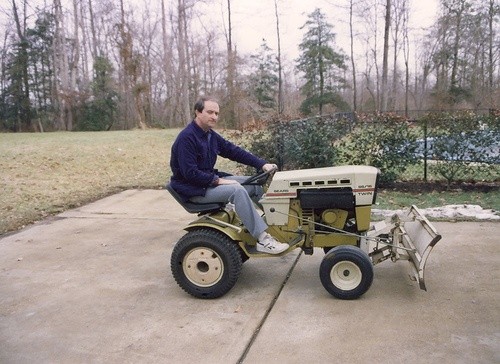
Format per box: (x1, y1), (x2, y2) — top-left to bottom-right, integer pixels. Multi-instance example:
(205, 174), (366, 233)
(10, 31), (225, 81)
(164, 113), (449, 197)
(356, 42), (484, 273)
(165, 164), (442, 300)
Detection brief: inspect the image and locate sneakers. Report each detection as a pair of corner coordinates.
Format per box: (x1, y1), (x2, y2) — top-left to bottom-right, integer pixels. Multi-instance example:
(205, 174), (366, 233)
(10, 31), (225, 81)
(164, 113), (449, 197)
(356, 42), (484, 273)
(257, 234), (289, 254)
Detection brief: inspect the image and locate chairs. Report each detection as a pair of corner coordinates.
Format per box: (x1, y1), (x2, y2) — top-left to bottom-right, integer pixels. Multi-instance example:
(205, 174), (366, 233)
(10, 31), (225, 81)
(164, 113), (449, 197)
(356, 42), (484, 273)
(166, 184), (226, 213)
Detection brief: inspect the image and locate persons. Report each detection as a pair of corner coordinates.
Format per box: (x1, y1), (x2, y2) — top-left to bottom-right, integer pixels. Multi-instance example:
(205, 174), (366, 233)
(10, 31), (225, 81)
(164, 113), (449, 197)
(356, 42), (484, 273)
(170, 98), (289, 254)
